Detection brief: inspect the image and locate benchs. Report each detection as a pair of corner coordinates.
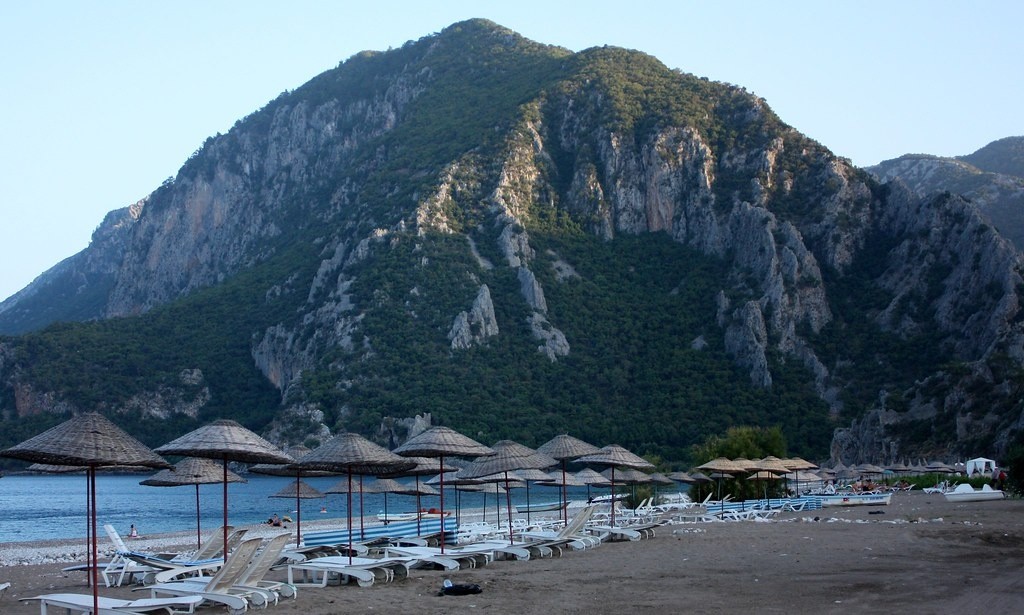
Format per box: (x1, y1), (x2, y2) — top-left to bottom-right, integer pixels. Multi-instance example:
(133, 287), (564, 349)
(17, 594), (206, 615)
(61, 490), (809, 614)
(801, 479), (959, 497)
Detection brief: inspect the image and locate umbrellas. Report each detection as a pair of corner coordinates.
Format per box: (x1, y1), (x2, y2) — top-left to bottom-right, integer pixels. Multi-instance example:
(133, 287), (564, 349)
(0, 410), (178, 615)
(259, 430), (1010, 528)
(150, 413), (295, 566)
(139, 456), (249, 558)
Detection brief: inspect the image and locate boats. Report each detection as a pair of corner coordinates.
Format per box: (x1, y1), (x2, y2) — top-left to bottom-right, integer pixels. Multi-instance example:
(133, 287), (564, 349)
(923, 487), (943, 493)
(516, 501), (570, 512)
(943, 484), (1005, 502)
(593, 494), (625, 502)
(376, 508), (451, 525)
(800, 492), (893, 507)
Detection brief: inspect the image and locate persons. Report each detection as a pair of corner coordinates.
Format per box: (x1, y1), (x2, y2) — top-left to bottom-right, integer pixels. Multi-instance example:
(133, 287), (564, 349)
(127, 523), (137, 539)
